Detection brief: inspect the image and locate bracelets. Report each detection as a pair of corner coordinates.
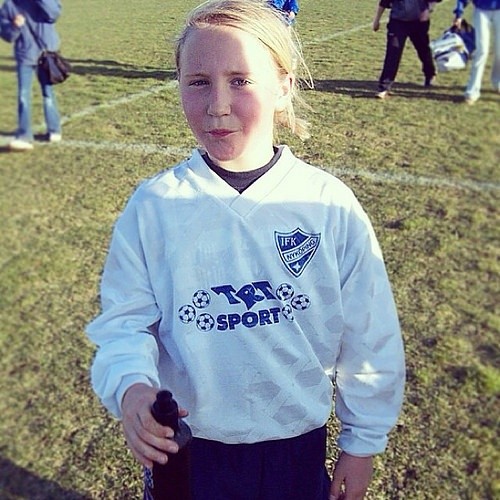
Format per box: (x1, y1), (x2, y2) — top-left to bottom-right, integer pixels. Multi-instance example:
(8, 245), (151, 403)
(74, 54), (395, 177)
(426, 7), (434, 13)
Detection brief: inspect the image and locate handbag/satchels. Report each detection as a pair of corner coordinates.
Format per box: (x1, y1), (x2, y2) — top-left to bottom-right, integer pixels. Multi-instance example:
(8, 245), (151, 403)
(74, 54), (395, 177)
(429, 18), (475, 75)
(38, 50), (71, 85)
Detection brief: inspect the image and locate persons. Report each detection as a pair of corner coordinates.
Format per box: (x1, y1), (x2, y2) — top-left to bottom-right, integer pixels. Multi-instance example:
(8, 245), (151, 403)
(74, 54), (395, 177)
(0, 0), (63, 150)
(372, 0), (444, 99)
(85, 0), (406, 500)
(452, 0), (500, 106)
(267, 0), (299, 32)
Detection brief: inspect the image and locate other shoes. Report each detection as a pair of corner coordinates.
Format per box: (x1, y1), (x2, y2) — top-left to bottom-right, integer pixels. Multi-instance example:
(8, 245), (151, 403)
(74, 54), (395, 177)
(425, 71), (437, 87)
(375, 86), (389, 98)
(9, 139), (35, 150)
(47, 132), (61, 142)
